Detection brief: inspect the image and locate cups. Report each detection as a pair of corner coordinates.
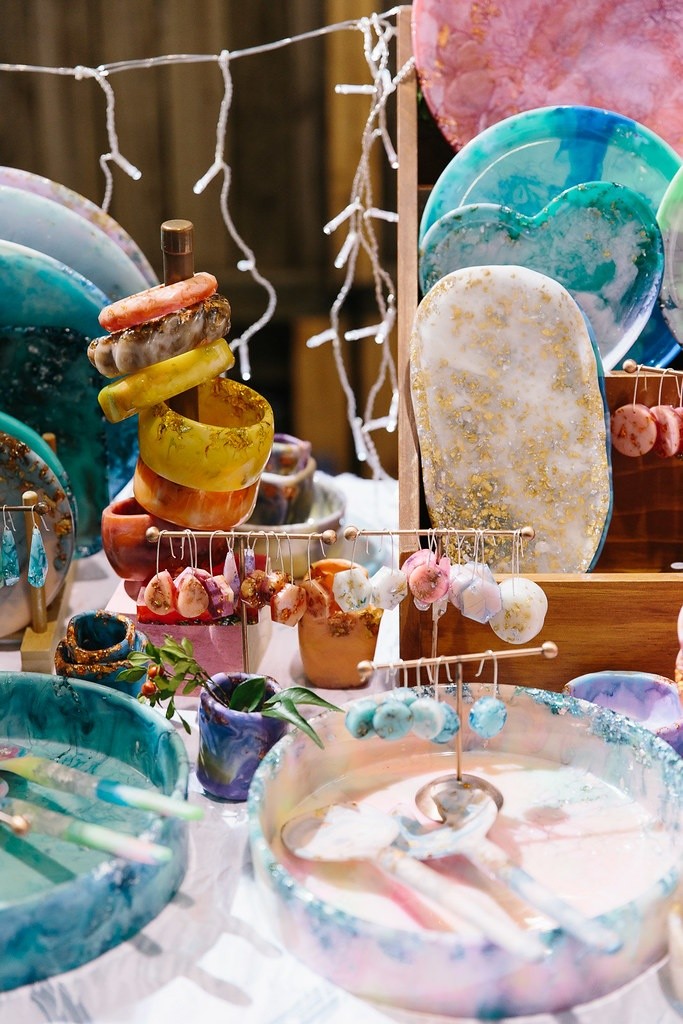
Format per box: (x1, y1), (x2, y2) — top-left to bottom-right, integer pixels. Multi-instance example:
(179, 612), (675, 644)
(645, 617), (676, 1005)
(196, 672), (290, 800)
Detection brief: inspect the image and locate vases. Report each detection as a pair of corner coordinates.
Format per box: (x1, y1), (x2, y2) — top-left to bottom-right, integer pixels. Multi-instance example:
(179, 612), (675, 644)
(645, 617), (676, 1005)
(195, 669), (290, 800)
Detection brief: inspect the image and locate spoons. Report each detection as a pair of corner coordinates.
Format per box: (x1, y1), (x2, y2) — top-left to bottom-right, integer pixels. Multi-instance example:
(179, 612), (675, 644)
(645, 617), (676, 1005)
(397, 794), (624, 952)
(285, 810), (550, 967)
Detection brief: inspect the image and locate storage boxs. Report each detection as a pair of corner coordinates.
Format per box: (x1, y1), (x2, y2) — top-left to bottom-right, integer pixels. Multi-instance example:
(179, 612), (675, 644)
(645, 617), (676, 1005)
(394, 0), (683, 696)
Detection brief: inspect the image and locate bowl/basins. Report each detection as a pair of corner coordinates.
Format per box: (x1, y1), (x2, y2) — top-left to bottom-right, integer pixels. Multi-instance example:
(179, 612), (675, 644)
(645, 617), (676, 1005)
(102, 498), (228, 605)
(231, 481), (346, 579)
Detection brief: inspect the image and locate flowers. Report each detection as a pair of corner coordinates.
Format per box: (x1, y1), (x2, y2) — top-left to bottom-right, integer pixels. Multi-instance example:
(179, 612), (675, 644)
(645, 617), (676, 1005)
(116, 634), (345, 750)
